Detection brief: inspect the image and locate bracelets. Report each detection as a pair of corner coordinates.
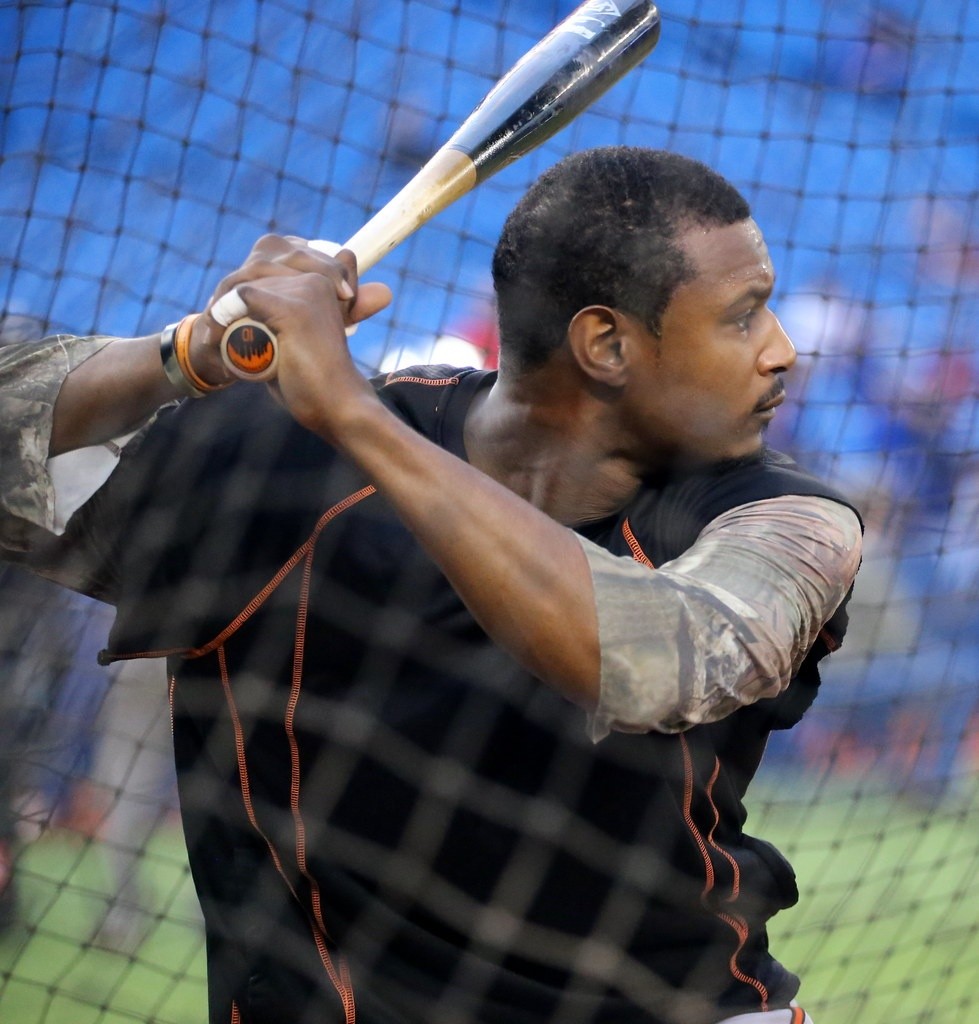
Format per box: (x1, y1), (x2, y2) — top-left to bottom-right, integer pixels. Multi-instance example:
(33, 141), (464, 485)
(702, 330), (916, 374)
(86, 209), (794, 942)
(159, 313), (238, 398)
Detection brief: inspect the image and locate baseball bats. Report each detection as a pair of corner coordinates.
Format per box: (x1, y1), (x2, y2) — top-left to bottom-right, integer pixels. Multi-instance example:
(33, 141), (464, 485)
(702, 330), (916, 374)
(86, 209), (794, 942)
(216, 1), (664, 387)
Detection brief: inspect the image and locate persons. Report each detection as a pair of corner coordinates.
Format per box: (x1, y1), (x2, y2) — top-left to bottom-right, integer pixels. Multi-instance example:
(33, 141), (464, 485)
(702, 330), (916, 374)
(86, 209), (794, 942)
(0, 145), (866, 1024)
